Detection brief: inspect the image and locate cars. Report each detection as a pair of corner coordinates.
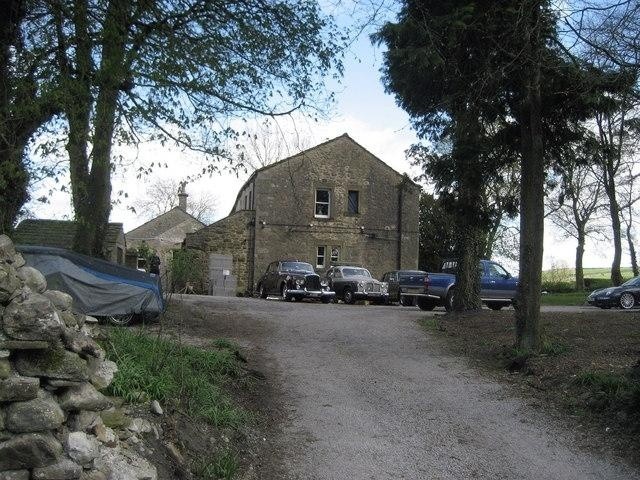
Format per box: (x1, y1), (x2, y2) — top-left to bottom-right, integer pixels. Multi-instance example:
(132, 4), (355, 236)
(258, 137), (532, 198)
(108, 314), (135, 325)
(257, 258), (519, 312)
(587, 274), (640, 309)
